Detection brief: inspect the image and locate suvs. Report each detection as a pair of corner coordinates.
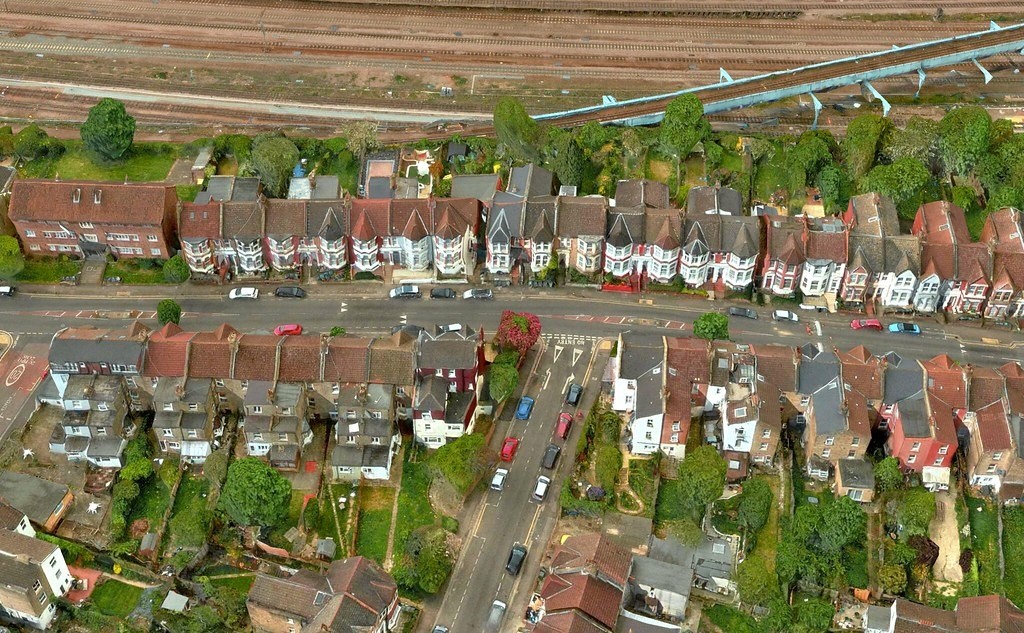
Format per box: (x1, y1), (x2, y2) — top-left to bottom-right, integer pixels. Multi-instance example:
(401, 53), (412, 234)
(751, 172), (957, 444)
(389, 286), (422, 299)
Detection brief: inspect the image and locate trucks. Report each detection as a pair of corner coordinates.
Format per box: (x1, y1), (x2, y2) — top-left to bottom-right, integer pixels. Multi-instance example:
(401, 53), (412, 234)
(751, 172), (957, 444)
(0, 285), (16, 297)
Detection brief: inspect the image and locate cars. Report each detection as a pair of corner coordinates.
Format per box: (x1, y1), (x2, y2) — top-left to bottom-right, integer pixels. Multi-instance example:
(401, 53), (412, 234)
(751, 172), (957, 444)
(515, 396), (534, 420)
(485, 600), (507, 631)
(565, 383), (583, 406)
(554, 412), (573, 440)
(462, 288), (494, 299)
(273, 323), (303, 336)
(531, 475), (551, 501)
(506, 546), (527, 576)
(430, 287), (456, 299)
(390, 325), (425, 337)
(541, 443), (561, 469)
(438, 323), (462, 332)
(729, 306), (758, 320)
(887, 322), (921, 334)
(850, 318), (883, 331)
(432, 624), (449, 633)
(771, 310), (799, 322)
(228, 286), (259, 300)
(490, 468), (509, 491)
(275, 286), (306, 298)
(500, 437), (518, 462)
